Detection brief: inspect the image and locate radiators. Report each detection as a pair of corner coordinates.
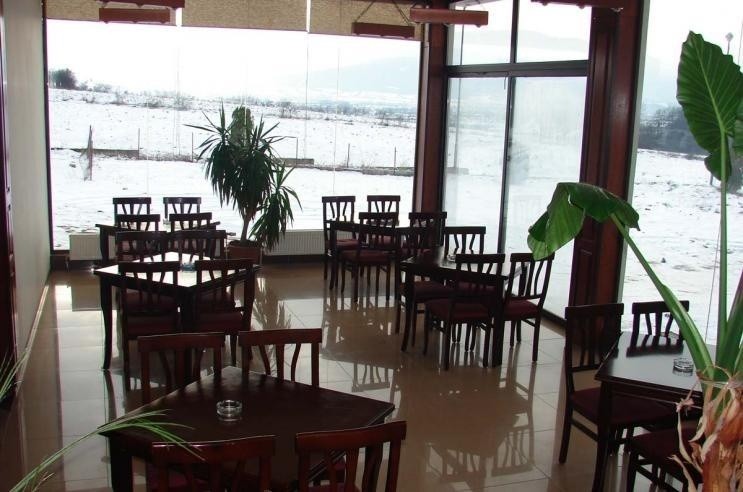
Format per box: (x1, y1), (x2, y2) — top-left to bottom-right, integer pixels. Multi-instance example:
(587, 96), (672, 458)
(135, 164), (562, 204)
(69, 233), (117, 262)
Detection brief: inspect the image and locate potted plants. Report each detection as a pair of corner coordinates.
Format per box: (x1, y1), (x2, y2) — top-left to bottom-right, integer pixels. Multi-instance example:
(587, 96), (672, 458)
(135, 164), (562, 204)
(183, 93), (304, 264)
